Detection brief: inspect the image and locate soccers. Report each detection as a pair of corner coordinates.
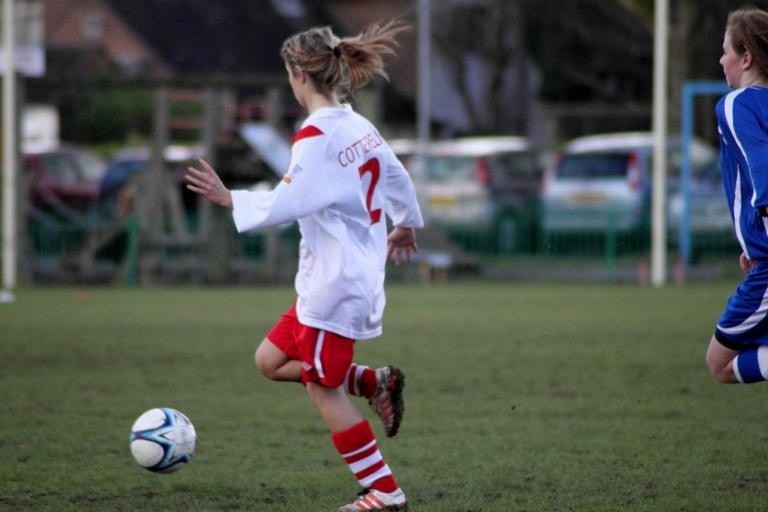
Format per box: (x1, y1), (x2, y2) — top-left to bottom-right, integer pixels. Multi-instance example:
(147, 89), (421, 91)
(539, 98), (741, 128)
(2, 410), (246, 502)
(130, 407), (196, 474)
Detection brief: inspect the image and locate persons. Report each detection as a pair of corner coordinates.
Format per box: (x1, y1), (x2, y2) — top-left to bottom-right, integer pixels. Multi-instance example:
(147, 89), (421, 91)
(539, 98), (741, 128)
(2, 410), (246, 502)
(183, 26), (426, 512)
(705, 9), (768, 385)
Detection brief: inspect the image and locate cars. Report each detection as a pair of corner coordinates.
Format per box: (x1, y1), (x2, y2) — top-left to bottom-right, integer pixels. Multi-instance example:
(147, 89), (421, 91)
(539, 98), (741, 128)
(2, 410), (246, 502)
(22, 140), (294, 262)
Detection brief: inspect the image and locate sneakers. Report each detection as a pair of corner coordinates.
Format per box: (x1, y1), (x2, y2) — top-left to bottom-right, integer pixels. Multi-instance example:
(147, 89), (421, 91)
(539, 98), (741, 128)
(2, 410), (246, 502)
(367, 366), (405, 437)
(337, 486), (409, 512)
